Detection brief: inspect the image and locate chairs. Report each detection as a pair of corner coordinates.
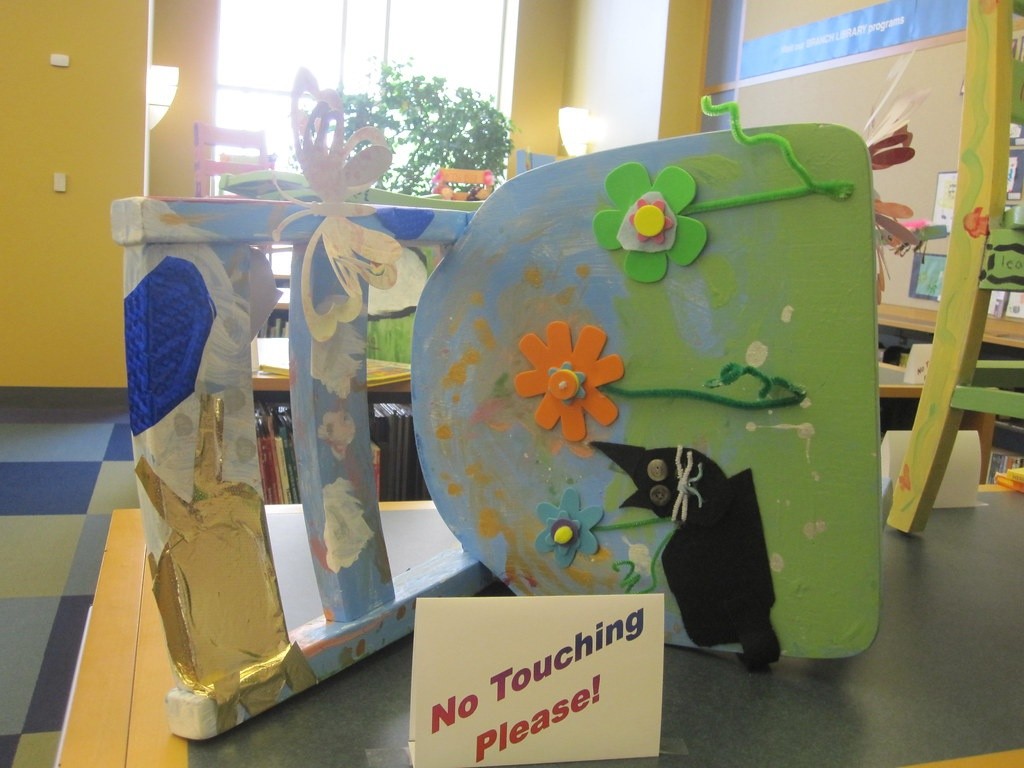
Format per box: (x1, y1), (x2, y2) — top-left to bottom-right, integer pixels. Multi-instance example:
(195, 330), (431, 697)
(114, 121), (886, 744)
(884, 0), (1024, 535)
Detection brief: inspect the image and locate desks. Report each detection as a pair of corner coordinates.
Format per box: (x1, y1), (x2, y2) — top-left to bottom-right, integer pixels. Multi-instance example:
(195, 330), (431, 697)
(53, 479), (1024, 767)
(267, 285), (292, 315)
(876, 301), (1023, 459)
(245, 351), (414, 395)
(874, 357), (999, 486)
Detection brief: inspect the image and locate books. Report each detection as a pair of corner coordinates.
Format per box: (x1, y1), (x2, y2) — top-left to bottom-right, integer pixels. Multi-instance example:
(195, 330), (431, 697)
(982, 445), (1024, 502)
(240, 310), (438, 506)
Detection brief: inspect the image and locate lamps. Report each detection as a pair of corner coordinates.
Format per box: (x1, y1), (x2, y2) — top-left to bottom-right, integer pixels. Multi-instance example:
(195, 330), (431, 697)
(145, 63), (180, 133)
(558, 106), (592, 159)
